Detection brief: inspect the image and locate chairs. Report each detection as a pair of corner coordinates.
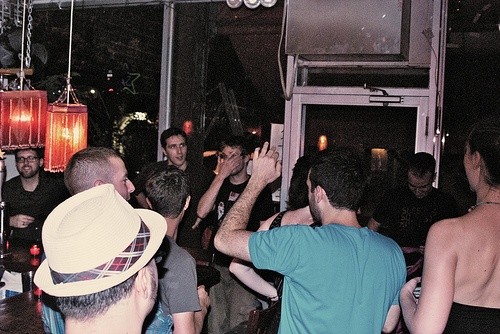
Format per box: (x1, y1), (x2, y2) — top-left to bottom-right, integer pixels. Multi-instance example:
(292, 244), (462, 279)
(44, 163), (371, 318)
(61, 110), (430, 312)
(246, 298), (282, 334)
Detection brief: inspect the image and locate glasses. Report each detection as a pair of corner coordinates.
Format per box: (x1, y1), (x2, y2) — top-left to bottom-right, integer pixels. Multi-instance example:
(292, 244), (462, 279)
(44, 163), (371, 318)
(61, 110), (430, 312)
(16, 156), (40, 163)
(219, 154), (242, 160)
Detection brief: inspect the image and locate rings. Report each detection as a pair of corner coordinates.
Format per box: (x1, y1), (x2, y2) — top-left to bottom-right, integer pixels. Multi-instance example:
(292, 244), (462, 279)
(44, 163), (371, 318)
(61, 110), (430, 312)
(21, 222), (24, 226)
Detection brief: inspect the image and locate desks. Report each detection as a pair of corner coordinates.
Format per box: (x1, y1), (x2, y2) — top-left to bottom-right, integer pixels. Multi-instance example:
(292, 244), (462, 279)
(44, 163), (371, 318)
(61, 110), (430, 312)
(0, 290), (45, 334)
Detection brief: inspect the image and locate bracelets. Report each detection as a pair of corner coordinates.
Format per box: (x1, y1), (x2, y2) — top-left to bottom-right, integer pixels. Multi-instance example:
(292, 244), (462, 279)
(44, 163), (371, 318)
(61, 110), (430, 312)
(268, 296), (278, 299)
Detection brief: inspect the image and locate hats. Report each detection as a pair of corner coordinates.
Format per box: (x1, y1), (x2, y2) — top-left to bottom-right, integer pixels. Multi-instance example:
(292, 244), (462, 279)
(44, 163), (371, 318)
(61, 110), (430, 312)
(33, 184), (167, 297)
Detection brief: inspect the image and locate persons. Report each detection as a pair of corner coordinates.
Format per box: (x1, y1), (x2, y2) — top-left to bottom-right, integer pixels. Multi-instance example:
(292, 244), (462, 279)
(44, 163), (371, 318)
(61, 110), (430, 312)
(0, 127), (458, 334)
(400, 115), (500, 334)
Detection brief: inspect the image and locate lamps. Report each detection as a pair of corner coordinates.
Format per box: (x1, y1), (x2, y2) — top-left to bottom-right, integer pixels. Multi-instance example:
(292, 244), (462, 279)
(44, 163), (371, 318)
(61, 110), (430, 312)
(0, 0), (48, 150)
(44, 0), (87, 173)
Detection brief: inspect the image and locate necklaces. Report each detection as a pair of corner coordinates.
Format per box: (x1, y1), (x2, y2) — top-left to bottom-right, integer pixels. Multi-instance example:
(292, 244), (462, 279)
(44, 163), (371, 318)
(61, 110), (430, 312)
(469, 202), (500, 211)
